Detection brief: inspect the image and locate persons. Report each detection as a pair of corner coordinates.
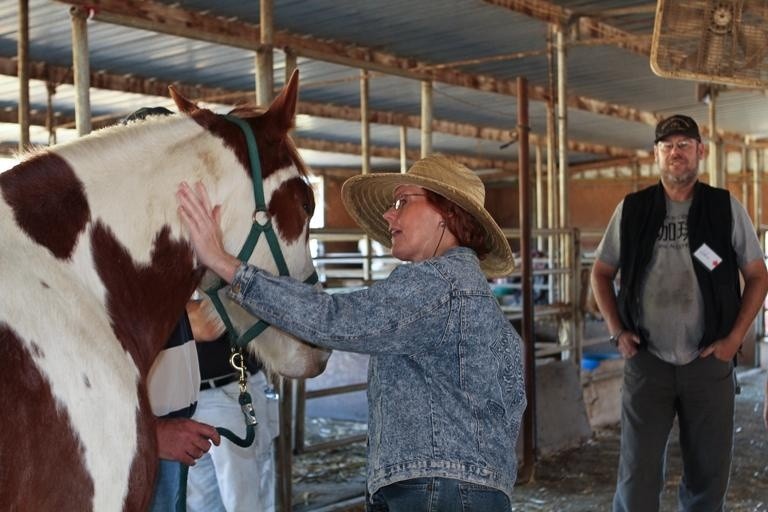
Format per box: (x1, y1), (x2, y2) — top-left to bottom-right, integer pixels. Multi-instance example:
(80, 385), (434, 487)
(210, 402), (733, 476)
(145, 305), (221, 512)
(588, 113), (766, 512)
(185, 289), (277, 512)
(174, 150), (527, 512)
(508, 252), (550, 307)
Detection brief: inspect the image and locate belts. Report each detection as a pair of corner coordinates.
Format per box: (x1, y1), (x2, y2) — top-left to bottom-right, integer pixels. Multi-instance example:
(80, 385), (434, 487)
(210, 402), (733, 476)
(199, 374), (248, 393)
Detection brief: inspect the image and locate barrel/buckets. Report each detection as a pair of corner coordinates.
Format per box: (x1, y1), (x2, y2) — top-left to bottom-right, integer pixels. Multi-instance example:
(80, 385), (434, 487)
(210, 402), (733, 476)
(582, 350), (620, 369)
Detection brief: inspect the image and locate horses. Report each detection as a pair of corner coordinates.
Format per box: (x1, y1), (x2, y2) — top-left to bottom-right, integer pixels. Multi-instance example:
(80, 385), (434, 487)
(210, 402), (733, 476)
(0, 67), (333, 512)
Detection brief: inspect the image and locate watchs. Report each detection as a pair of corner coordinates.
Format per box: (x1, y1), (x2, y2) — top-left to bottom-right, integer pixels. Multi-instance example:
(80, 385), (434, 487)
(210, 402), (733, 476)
(608, 327), (623, 348)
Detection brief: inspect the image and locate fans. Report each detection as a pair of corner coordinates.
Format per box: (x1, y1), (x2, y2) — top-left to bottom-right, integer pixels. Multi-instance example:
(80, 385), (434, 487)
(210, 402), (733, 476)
(648, 0), (767, 94)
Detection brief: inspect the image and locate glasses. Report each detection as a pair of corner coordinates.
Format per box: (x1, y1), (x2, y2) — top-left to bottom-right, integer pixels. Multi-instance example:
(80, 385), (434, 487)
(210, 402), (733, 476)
(385, 187), (432, 212)
(656, 141), (699, 151)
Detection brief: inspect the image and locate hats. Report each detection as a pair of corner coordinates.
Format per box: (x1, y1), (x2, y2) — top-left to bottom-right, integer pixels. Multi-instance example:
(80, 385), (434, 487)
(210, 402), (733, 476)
(341, 151), (516, 283)
(654, 115), (701, 145)
